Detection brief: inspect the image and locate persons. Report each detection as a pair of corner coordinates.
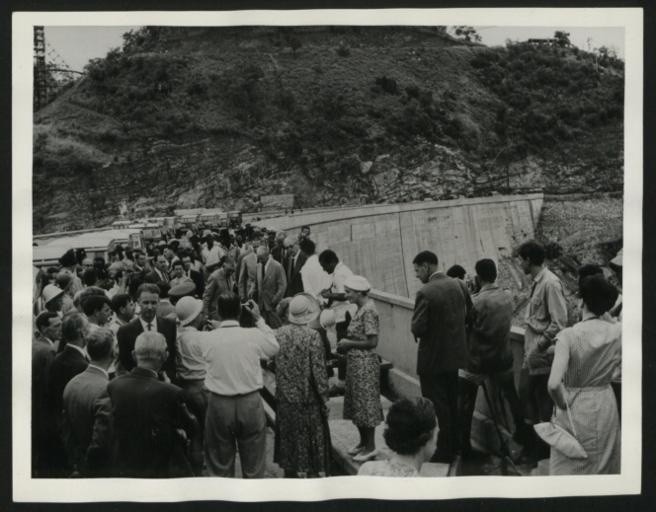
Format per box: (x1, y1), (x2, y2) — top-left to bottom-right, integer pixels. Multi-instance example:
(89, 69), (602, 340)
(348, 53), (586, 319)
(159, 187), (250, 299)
(33, 217), (382, 478)
(408, 251), (465, 463)
(358, 395), (440, 477)
(448, 239), (623, 474)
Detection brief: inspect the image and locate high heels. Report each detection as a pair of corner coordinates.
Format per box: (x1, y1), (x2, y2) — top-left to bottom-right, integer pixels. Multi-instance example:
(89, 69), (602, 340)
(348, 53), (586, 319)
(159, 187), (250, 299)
(352, 446), (378, 462)
(347, 442), (365, 456)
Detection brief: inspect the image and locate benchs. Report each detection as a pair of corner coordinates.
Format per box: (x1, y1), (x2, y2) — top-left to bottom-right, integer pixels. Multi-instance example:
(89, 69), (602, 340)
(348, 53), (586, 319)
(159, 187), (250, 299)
(381, 357), (398, 389)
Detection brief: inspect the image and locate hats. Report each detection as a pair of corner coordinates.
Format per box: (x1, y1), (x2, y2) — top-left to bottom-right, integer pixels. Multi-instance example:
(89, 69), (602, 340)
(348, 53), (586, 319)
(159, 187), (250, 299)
(175, 297), (205, 326)
(288, 292), (321, 325)
(55, 269), (75, 290)
(343, 274), (371, 293)
(43, 285), (65, 307)
(168, 281), (197, 301)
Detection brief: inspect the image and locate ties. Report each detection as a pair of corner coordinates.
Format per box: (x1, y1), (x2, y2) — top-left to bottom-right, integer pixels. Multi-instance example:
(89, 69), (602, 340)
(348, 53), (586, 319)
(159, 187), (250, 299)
(263, 263), (265, 279)
(226, 279), (231, 290)
(148, 321), (152, 330)
(291, 256), (294, 273)
(160, 271), (167, 282)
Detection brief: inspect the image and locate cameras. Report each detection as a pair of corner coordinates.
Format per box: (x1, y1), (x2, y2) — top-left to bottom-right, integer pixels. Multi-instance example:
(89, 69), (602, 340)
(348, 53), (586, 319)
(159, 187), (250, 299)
(241, 304), (252, 310)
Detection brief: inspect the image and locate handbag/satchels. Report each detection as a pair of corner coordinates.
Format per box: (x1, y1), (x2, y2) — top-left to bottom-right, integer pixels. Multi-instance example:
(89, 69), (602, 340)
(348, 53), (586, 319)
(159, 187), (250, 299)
(533, 423), (589, 460)
